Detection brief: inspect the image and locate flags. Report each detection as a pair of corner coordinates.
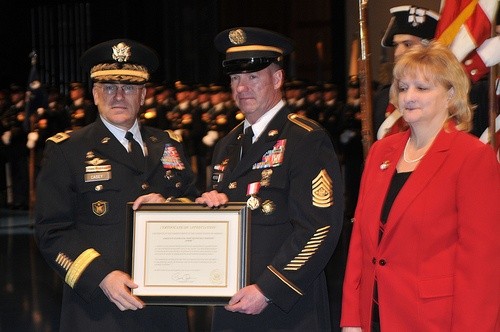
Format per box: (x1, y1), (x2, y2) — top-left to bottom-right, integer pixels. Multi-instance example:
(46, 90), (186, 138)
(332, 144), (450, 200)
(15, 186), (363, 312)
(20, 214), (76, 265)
(377, 0), (499, 139)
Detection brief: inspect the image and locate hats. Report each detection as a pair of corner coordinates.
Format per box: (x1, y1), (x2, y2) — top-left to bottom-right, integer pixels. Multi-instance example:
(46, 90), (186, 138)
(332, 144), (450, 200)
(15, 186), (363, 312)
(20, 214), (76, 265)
(381, 4), (442, 51)
(0, 72), (362, 92)
(215, 26), (293, 75)
(80, 38), (164, 85)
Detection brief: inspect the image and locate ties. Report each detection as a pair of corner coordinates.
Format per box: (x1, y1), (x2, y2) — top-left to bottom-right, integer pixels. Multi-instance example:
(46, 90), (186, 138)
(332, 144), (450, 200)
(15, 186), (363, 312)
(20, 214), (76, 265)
(239, 125), (255, 161)
(123, 130), (148, 175)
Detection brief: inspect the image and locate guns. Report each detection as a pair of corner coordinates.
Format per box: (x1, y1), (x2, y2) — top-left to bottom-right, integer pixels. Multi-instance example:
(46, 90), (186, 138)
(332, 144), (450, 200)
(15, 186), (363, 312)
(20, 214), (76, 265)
(357, 1), (374, 157)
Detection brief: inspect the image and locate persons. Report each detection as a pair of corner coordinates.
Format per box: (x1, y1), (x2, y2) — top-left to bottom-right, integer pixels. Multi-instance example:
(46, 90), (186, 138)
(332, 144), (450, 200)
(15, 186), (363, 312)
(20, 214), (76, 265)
(0, 82), (361, 211)
(35, 42), (198, 332)
(194, 27), (345, 332)
(340, 42), (500, 332)
(375, 6), (484, 134)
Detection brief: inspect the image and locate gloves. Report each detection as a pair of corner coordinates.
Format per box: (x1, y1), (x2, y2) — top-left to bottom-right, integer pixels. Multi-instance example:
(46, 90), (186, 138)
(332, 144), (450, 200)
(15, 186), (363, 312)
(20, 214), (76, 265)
(2, 130), (11, 145)
(202, 130), (219, 146)
(174, 129), (184, 136)
(26, 132), (39, 148)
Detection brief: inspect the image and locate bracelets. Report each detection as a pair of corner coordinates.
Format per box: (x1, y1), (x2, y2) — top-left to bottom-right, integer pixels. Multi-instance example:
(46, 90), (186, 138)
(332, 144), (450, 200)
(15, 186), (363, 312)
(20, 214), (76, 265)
(265, 295), (272, 303)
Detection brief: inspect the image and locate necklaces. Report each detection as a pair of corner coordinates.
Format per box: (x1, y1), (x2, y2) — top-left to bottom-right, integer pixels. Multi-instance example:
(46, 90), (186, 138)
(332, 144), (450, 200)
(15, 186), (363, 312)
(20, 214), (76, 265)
(403, 137), (423, 163)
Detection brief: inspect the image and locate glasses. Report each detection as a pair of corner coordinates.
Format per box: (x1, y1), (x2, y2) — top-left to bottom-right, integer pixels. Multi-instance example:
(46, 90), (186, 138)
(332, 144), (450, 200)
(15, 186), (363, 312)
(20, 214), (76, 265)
(92, 81), (147, 94)
